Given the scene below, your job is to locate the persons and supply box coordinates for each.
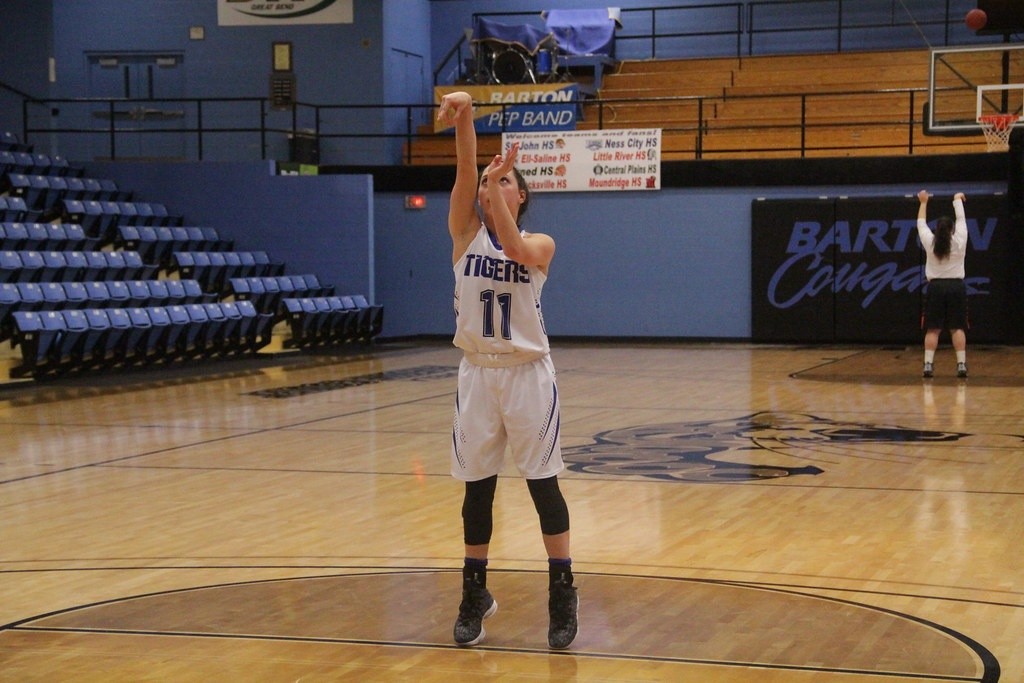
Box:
[434,91,579,647]
[918,190,968,378]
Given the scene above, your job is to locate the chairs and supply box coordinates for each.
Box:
[0,129,386,381]
[549,7,617,90]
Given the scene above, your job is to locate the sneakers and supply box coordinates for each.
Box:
[923,363,933,378]
[548,584,578,648]
[957,362,968,378]
[453,587,497,645]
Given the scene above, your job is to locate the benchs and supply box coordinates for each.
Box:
[399,49,1024,166]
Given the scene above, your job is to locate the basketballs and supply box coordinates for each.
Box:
[966,9,987,30]
